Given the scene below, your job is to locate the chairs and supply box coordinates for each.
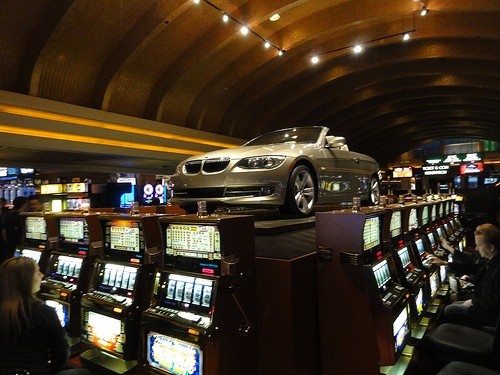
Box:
[428,313,500,375]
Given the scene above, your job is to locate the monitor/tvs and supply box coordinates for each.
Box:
[24,216,49,242]
[56,217,91,244]
[141,327,205,375]
[89,259,141,299]
[163,222,224,272]
[13,245,45,268]
[100,218,144,260]
[80,307,128,356]
[157,267,215,312]
[38,295,71,328]
[363,175,498,362]
[48,251,84,282]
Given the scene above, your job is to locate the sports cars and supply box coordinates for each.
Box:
[170,125,382,218]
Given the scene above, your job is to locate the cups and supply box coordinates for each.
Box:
[132,202,139,216]
[353,197,360,211]
[379,193,447,208]
[197,200,207,218]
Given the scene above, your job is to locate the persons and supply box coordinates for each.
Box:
[0,196,42,257]
[426,223,500,327]
[0,257,92,375]
